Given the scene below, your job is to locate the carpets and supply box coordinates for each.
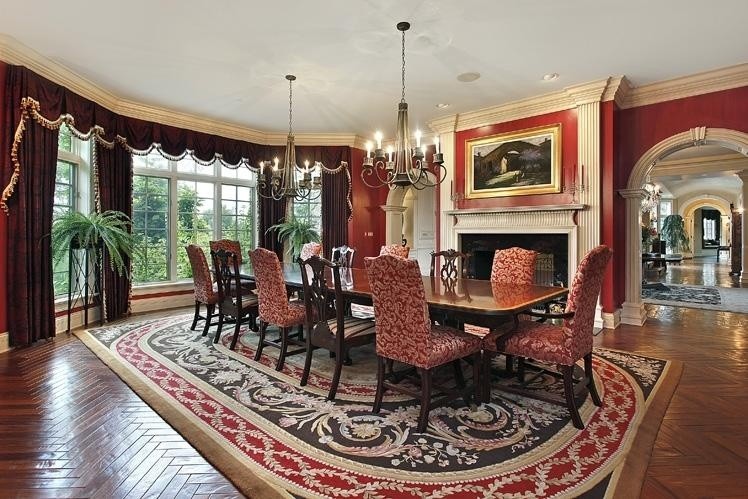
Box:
[641,282,748,314]
[72,311,684,499]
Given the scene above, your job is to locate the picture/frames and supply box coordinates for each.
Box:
[464,122,563,200]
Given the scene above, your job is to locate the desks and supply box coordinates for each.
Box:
[716,246,729,262]
[642,257,666,277]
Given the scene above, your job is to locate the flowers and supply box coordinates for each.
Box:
[649,228,657,236]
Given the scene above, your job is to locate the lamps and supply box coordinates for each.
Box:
[641,182,662,210]
[256,75,323,202]
[361,21,447,191]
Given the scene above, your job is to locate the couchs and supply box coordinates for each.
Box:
[664,253,682,266]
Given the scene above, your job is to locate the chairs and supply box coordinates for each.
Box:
[483,245,614,430]
[296,255,375,400]
[378,245,410,258]
[430,249,469,278]
[247,248,329,371]
[210,248,259,351]
[300,242,322,260]
[331,245,356,268]
[185,244,237,337]
[209,239,242,269]
[362,253,483,434]
[462,247,537,373]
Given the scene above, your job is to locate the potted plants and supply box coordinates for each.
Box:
[40,209,136,281]
